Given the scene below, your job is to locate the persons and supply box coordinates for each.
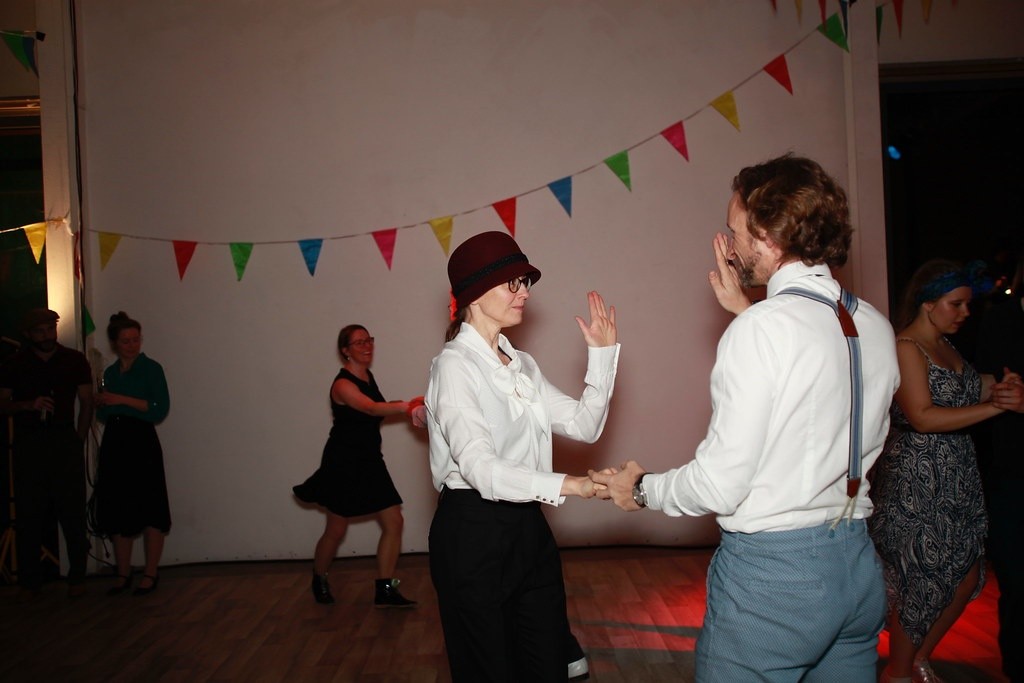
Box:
[292,324,429,609]
[0,311,172,602]
[870,258,1024,683]
[588,152,901,683]
[407,231,620,683]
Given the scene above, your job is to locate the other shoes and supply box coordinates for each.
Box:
[912,658,942,682]
[881,668,912,683]
[130,569,160,598]
[311,566,336,606]
[105,573,134,598]
[374,577,418,610]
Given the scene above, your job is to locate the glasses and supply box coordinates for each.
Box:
[348,336,375,349]
[508,276,532,293]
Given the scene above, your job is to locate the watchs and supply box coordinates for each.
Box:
[632,472,654,508]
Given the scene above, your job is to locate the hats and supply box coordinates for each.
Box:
[447,231,542,310]
